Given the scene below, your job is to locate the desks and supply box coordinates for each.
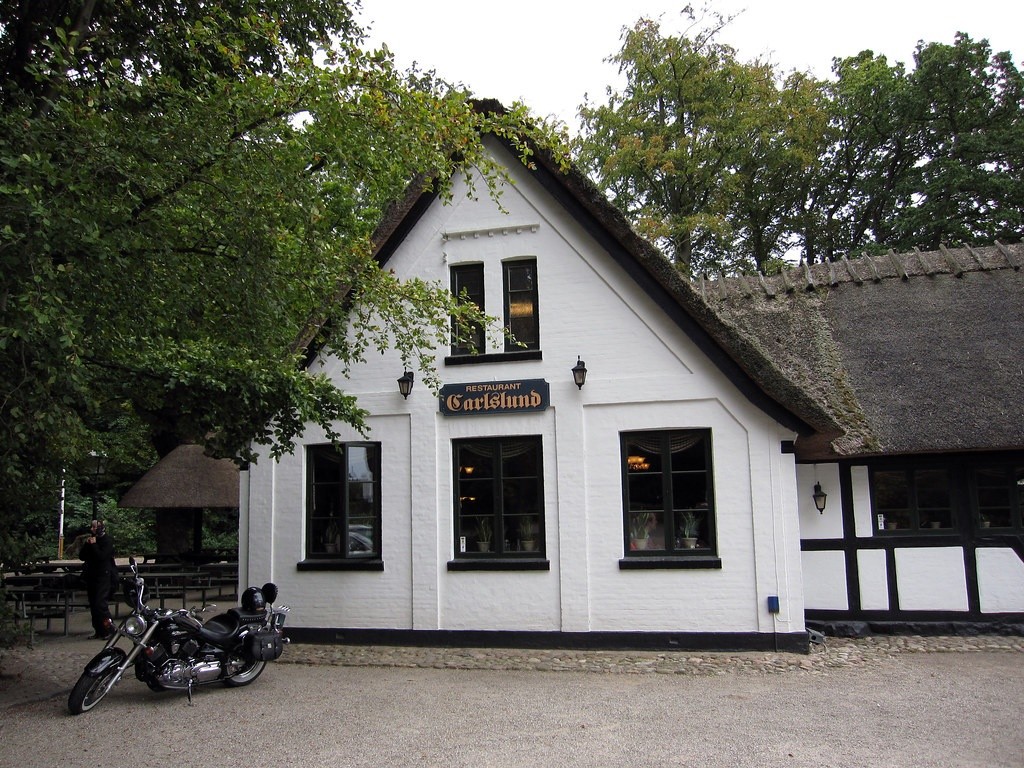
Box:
[4,588,75,642]
[116,563,182,573]
[118,570,211,609]
[200,562,238,601]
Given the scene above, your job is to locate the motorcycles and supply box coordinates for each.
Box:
[68,556,291,715]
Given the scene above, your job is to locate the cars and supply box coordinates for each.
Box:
[349,524,375,559]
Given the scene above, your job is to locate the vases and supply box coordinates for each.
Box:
[929,521,941,528]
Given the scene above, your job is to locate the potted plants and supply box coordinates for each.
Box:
[630,512,652,550]
[321,518,339,553]
[980,514,992,528]
[472,516,493,552]
[885,512,899,529]
[680,511,701,549]
[518,516,538,551]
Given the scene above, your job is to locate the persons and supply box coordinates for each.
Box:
[79,519,120,640]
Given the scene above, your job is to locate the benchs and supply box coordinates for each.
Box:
[0,553,239,641]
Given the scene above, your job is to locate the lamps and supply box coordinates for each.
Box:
[627,456,650,472]
[571,355,588,390]
[811,481,827,514]
[397,367,415,400]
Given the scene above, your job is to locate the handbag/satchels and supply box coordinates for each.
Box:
[244,629,283,662]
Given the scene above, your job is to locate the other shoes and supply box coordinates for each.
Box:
[88,633,102,640]
[103,632,115,640]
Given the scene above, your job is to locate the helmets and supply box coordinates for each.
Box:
[241,587,266,615]
[123,582,150,608]
